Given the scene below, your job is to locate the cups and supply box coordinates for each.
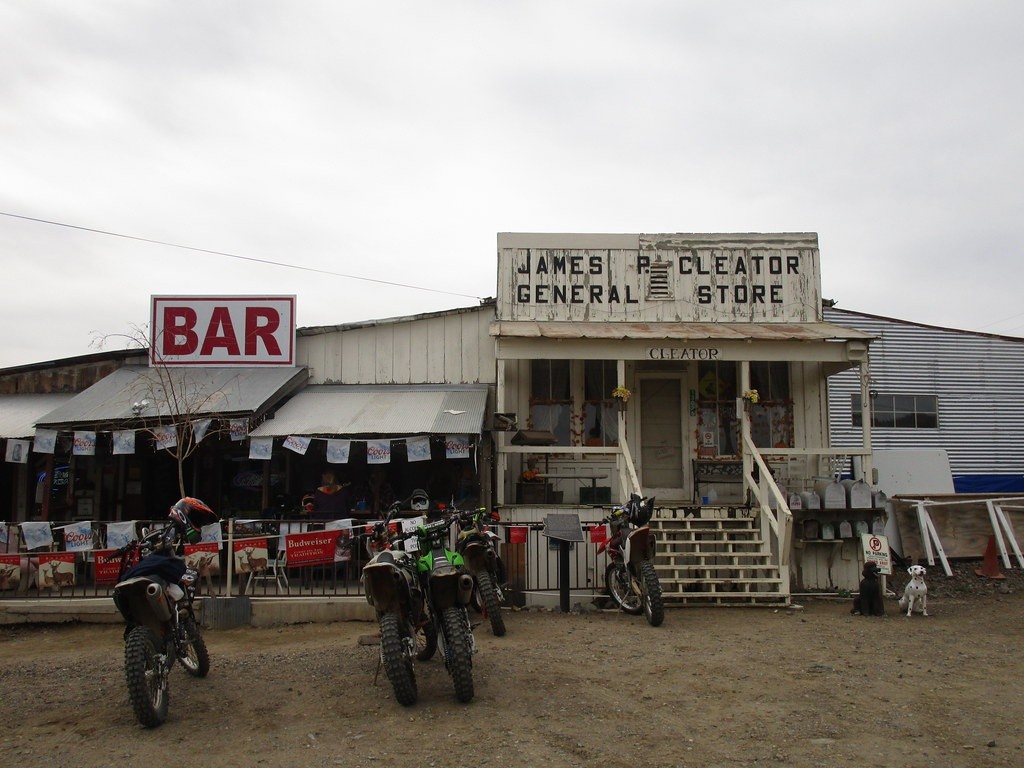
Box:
[702,497,709,504]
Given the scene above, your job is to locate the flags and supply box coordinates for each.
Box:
[406,436,431,462]
[445,434,469,458]
[65,522,93,551]
[21,522,52,550]
[249,436,391,464]
[277,519,425,553]
[200,523,223,550]
[5,417,248,464]
[106,521,132,549]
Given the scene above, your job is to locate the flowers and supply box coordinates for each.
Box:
[742,389,760,403]
[517,467,545,484]
[613,384,631,401]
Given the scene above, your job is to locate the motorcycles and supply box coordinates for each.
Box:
[355,505,507,705]
[102,519,209,730]
[149,627,151,628]
[596,494,666,627]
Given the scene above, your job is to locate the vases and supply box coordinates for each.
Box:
[618,399,627,411]
[579,487,611,505]
[516,483,554,504]
[744,399,752,413]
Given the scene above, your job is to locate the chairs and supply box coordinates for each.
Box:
[247,550,290,597]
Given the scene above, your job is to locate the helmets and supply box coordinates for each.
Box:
[302,494,316,511]
[410,489,430,511]
[275,492,295,512]
[335,534,349,548]
[168,496,217,546]
[630,494,656,529]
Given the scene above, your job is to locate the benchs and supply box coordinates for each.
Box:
[692,458,776,507]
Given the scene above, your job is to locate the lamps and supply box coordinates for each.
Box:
[132,399,150,414]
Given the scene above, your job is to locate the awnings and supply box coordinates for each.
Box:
[0,390,79,442]
[29,364,308,421]
[245,384,488,442]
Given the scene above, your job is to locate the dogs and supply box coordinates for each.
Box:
[851,560,884,616]
[898,565,931,617]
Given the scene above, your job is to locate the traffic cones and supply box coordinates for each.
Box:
[974,534,1007,580]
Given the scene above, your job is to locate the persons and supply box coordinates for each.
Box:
[312,471,356,519]
[457,463,473,496]
[371,471,397,510]
[586,428,603,446]
